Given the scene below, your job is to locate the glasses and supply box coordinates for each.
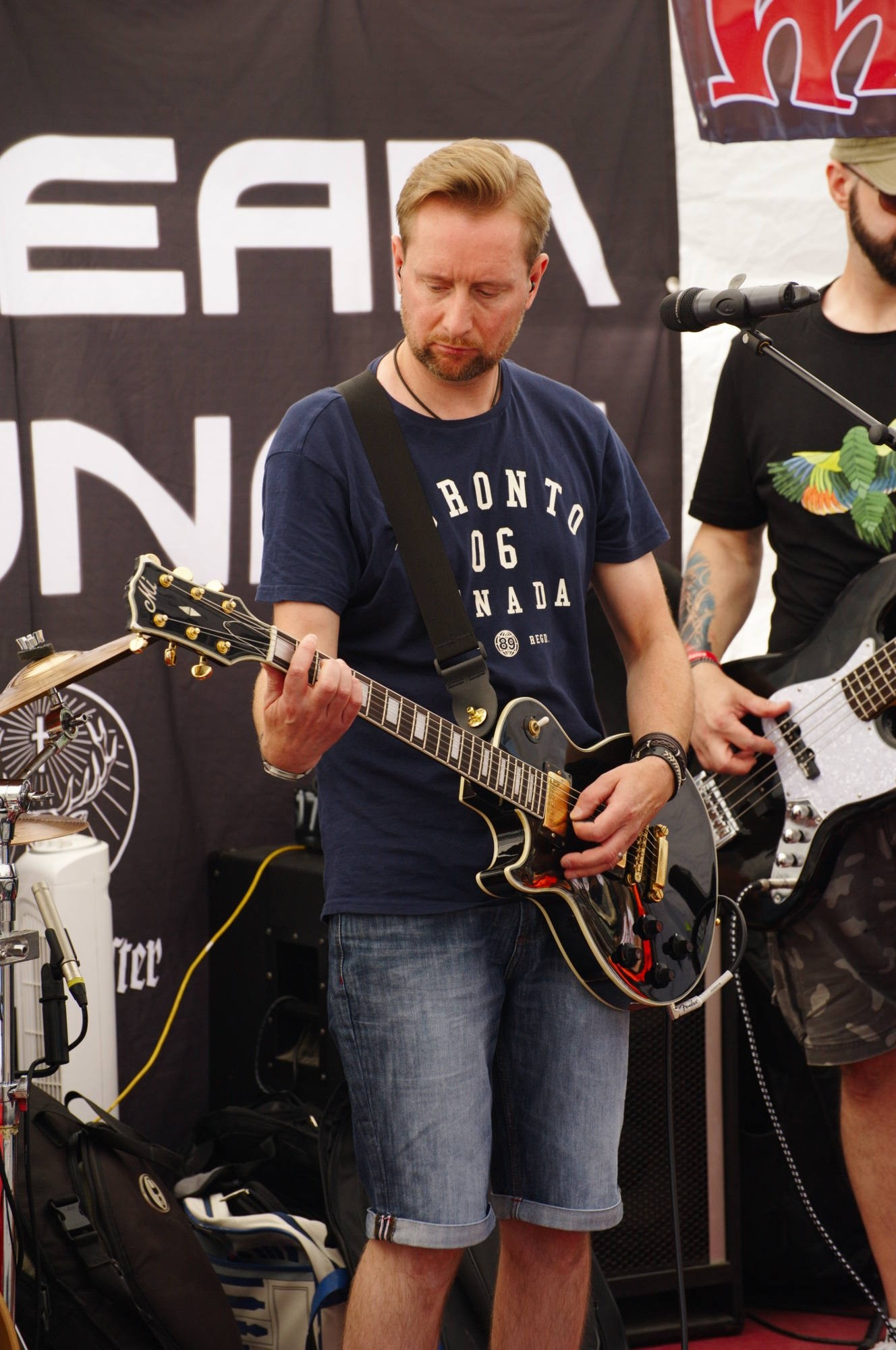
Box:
[840,163,896,215]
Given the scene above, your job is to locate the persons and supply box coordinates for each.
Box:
[255,139,702,1350]
[680,133,896,1350]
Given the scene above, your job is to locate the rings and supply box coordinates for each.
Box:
[617,851,624,860]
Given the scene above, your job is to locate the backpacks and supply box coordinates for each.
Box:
[170,1163,351,1350]
[8,1080,245,1350]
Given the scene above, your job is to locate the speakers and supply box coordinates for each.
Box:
[206,841,742,1299]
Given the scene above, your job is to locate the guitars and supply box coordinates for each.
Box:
[122,549,723,1005]
[713,532,896,934]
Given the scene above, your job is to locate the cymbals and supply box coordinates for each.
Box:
[9,811,87,846]
[2,629,157,714]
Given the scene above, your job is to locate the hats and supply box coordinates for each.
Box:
[828,137,896,197]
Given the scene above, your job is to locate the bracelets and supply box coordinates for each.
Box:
[688,649,720,666]
[632,733,686,802]
[263,758,317,782]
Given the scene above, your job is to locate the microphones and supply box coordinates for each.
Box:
[30,881,89,1008]
[658,281,822,334]
